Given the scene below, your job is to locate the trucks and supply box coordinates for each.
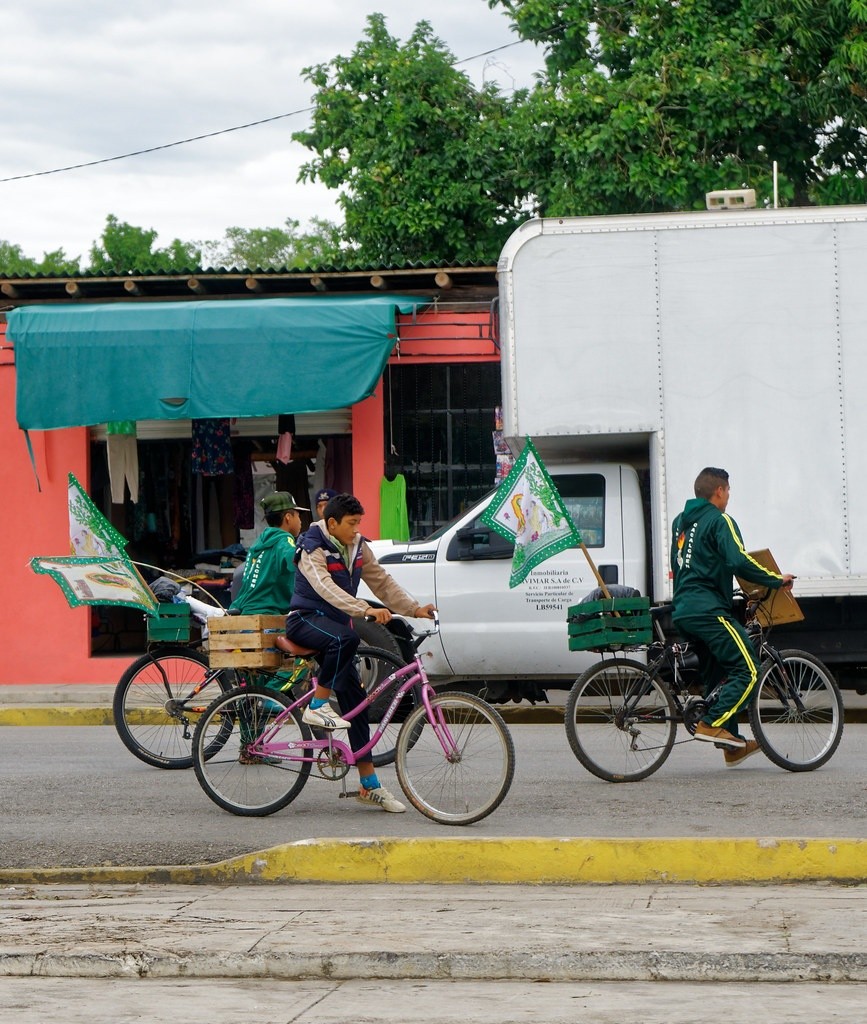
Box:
[286,188,866,721]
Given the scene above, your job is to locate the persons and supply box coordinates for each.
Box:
[228,491,311,765]
[284,492,440,813]
[315,488,337,519]
[670,467,795,767]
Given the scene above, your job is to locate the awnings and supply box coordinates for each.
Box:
[2,287,439,494]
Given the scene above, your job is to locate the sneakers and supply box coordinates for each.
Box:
[301,703,352,729]
[695,721,762,768]
[238,746,282,764]
[356,783,406,813]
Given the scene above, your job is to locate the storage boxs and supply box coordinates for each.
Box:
[206,614,289,667]
[567,596,651,651]
[146,604,191,641]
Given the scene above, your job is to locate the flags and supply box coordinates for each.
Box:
[29,556,161,621]
[479,436,583,590]
[67,471,130,558]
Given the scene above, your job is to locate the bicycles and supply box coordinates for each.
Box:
[111,643,425,772]
[190,608,517,826]
[562,575,847,783]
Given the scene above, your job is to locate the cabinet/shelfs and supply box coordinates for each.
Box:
[384,408,498,527]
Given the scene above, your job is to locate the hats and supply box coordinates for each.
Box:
[315,489,337,503]
[261,491,311,513]
